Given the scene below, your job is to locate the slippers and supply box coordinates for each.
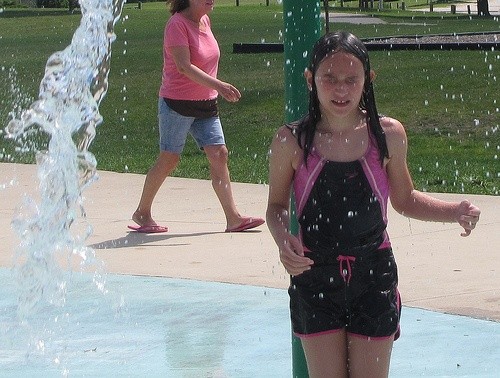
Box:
[127,225,168,232]
[224,215,266,232]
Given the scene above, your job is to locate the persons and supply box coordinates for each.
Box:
[265,30,480,378]
[127,0,264,234]
